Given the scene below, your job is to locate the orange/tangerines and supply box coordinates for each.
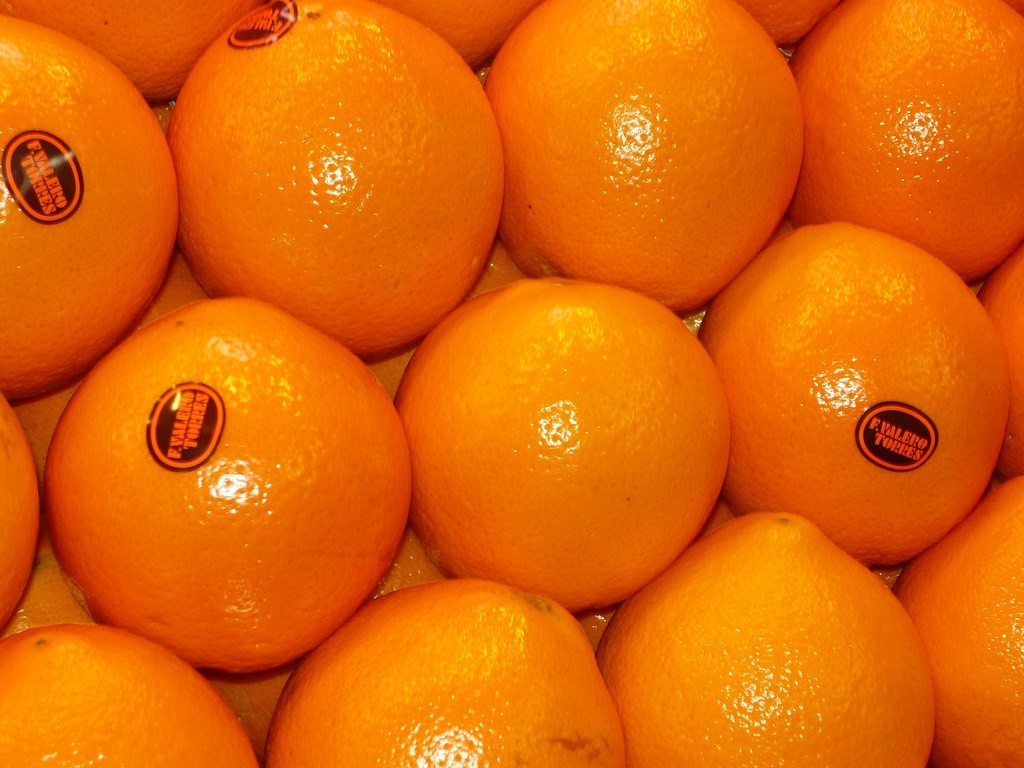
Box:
[0,0,1024,768]
